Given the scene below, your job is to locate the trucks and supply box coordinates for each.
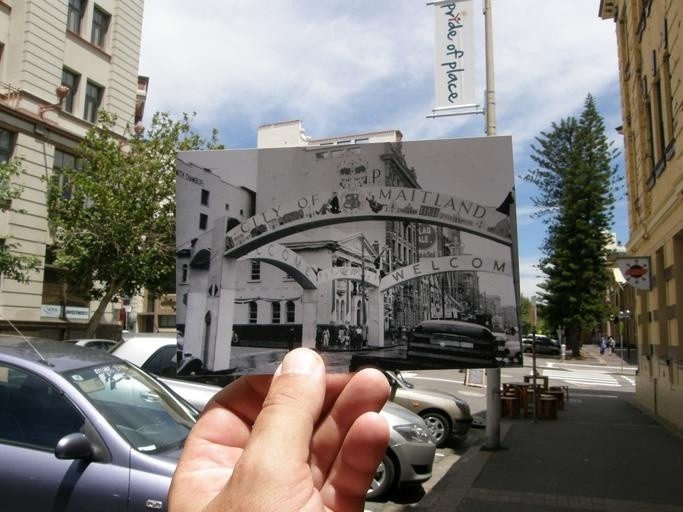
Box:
[348,322,510,371]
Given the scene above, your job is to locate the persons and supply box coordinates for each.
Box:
[318,324,362,346]
[598,334,616,357]
[165,348,390,512]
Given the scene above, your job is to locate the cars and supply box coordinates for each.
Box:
[523,333,559,355]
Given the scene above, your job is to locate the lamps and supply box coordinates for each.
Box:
[119,123,145,149]
[40,86,69,127]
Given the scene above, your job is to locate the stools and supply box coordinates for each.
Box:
[502,375,569,419]
